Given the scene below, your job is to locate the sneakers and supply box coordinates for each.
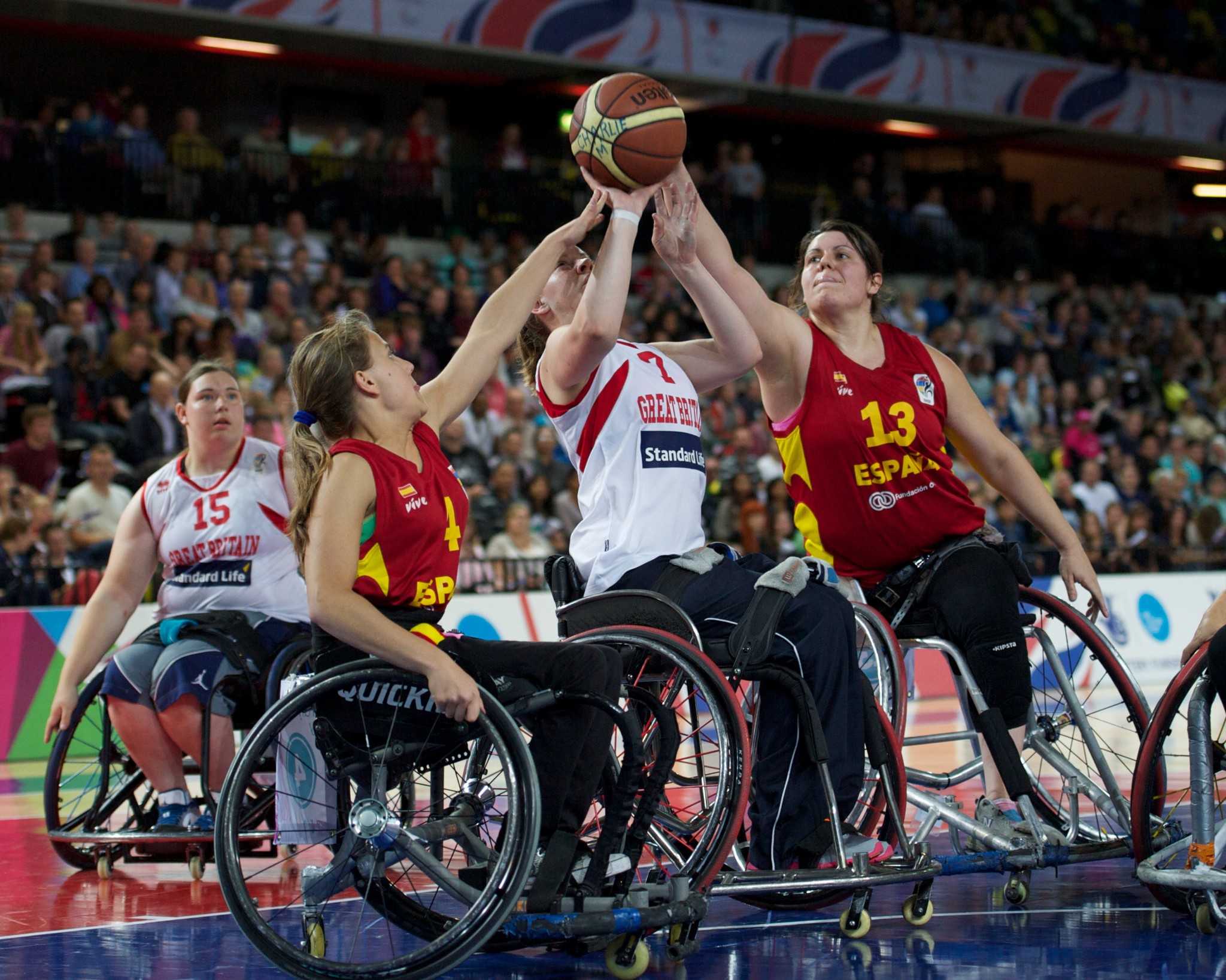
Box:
[486,846,631,892]
[968,793,1068,854]
[746,832,895,872]
[153,787,192,833]
[191,804,218,831]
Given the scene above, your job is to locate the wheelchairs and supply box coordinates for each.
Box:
[213,622,751,980]
[460,549,1226,980]
[44,612,312,881]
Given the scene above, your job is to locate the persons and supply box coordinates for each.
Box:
[1178,588,1226,663]
[289,187,606,903]
[660,159,1108,848]
[0,98,1226,589]
[518,166,892,866]
[44,364,311,831]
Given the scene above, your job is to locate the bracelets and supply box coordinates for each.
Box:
[609,209,640,226]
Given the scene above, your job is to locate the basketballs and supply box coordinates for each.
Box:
[568,73,687,191]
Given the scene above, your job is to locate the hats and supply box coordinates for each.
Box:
[1075,409,1093,423]
